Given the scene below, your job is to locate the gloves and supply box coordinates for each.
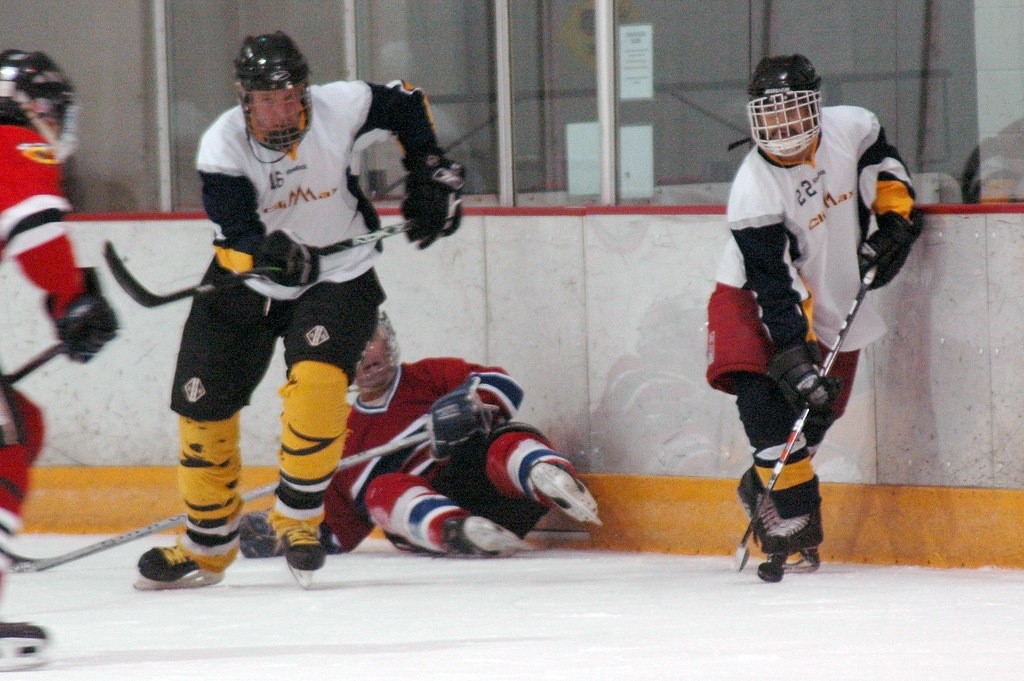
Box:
[426,386,490,460]
[859,211,923,290]
[44,267,119,363]
[400,147,465,250]
[253,228,320,288]
[767,345,842,425]
[239,507,286,558]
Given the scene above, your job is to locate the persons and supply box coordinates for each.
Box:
[240,311,598,557]
[961,118,1024,205]
[706,54,922,554]
[0,46,118,656]
[137,30,467,582]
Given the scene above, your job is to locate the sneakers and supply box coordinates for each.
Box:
[735,463,824,583]
[0,621,47,671]
[267,510,324,587]
[133,542,225,590]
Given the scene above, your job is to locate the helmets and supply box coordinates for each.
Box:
[234,30,312,147]
[747,54,821,156]
[0,50,74,126]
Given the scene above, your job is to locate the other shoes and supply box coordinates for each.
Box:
[530,463,604,526]
[447,516,535,557]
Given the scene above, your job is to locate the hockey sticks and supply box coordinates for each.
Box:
[103,216,407,308]
[735,261,880,572]
[1,333,74,391]
[0,402,485,575]
